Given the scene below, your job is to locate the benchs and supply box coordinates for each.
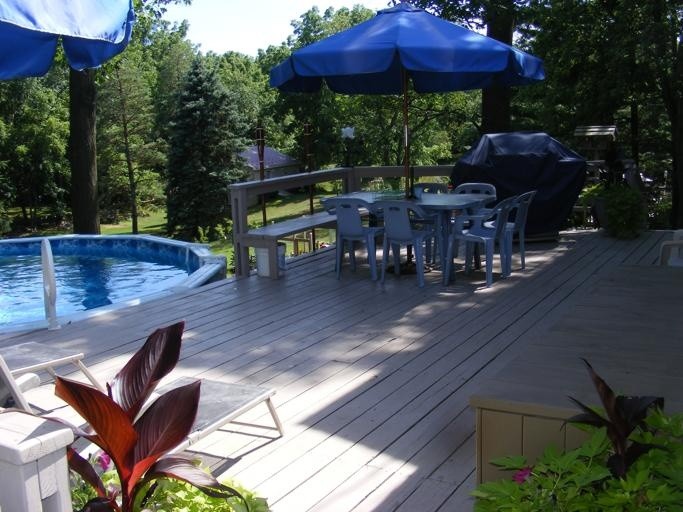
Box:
[238,207,370,280]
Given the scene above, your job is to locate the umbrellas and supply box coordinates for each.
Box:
[0,1,135,84]
[267,1,545,196]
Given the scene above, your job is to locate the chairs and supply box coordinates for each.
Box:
[0,341,284,458]
[319,183,537,289]
[658,229,683,267]
